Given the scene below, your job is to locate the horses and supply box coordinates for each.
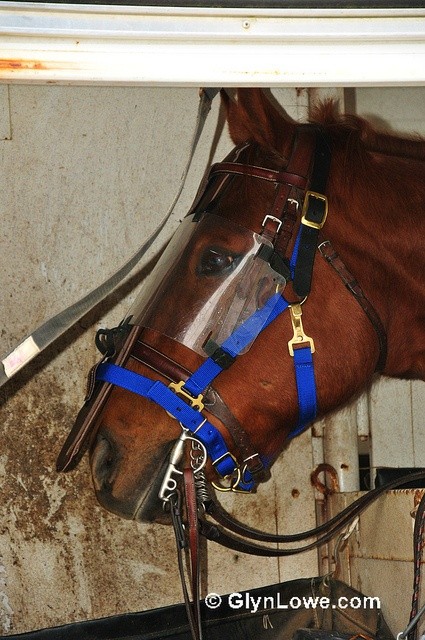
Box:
[84,86,425,527]
